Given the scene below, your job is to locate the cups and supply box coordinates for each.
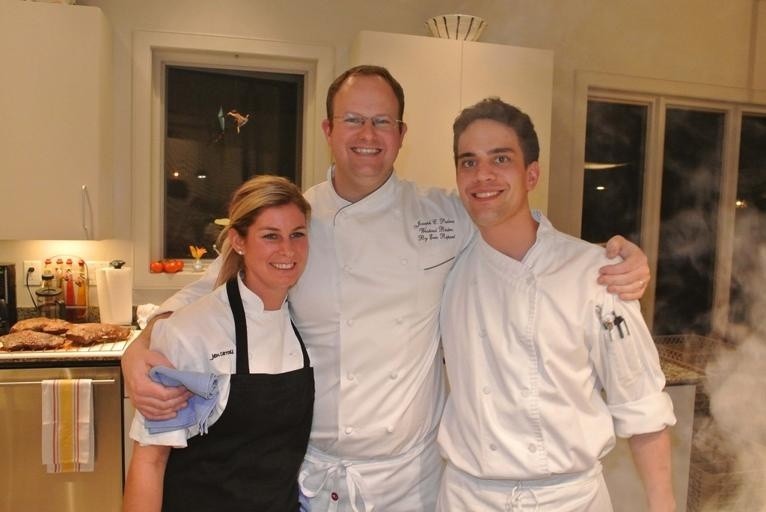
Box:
[36,287,67,320]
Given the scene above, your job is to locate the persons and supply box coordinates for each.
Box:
[118,63,652,511]
[118,174,316,510]
[434,96,680,511]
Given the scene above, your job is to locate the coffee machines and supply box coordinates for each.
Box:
[0,261,18,334]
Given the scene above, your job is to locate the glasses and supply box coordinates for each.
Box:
[331,110,403,130]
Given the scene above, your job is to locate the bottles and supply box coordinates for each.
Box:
[44,258,89,318]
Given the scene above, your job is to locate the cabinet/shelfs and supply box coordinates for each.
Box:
[0,358,136,510]
[0,2,116,243]
[334,29,555,218]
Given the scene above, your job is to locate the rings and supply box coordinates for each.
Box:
[638,278,646,289]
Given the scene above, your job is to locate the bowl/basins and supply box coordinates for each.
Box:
[423,13,489,42]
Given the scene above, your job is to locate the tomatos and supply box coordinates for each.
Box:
[164,261,178,273]
[173,260,184,270]
[151,261,163,273]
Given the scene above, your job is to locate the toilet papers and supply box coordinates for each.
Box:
[95,266,134,326]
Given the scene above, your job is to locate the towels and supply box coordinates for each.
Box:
[40,377,95,475]
